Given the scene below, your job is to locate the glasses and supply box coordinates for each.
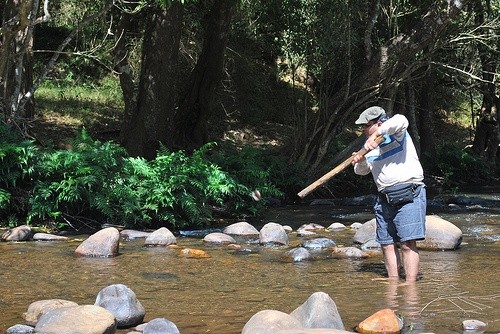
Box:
[359,122,371,128]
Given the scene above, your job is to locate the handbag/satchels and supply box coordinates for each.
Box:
[379,184,418,206]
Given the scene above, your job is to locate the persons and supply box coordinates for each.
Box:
[352,106,426,285]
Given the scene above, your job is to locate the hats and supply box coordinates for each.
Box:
[355,106,386,124]
